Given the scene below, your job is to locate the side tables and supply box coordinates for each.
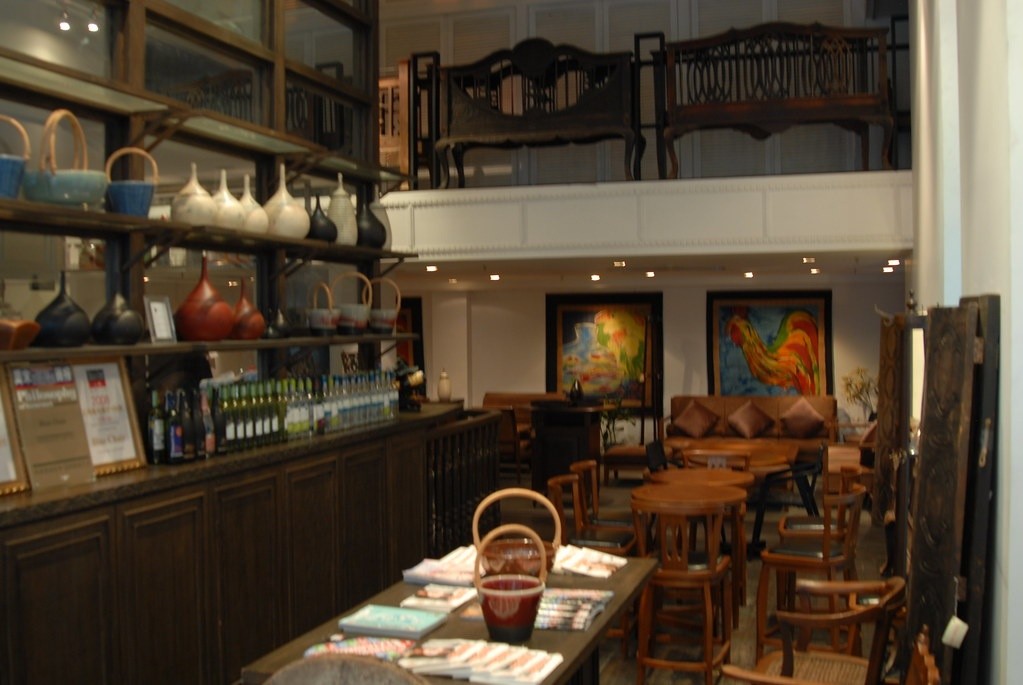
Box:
[840,433,861,443]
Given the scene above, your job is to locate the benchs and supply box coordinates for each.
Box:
[437,37,636,189]
[664,20,894,181]
[658,395,837,463]
[483,392,544,440]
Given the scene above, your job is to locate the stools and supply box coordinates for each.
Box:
[604,444,674,490]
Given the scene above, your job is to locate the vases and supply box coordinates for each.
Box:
[570,379,582,406]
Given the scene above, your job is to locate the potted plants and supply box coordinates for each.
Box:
[841,364,878,422]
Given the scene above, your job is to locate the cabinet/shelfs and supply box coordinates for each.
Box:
[532,404,604,507]
[0,196,419,358]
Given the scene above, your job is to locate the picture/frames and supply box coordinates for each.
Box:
[0,360,30,494]
[5,356,147,485]
[546,291,664,416]
[706,288,833,396]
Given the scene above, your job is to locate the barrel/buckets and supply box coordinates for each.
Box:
[303,271,401,337]
[472,488,562,577]
[472,523,547,643]
[0,106,160,219]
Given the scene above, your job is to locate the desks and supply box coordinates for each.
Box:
[240,556,660,685]
[629,484,748,629]
[677,441,799,512]
[651,467,756,488]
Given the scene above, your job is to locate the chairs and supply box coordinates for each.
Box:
[465,407,530,487]
[546,442,907,685]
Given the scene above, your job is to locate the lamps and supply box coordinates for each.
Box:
[60,5,71,32]
[87,6,99,34]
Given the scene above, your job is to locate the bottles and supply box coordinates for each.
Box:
[34,257,266,347]
[170,162,393,252]
[437,368,451,402]
[147,368,401,465]
[570,379,585,403]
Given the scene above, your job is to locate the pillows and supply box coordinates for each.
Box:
[672,399,719,438]
[779,397,823,438]
[728,398,773,439]
